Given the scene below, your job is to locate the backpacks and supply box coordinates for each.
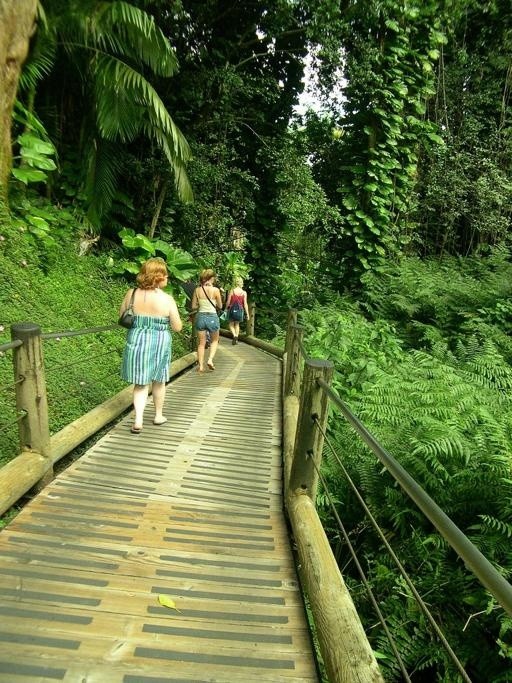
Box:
[230,301,244,321]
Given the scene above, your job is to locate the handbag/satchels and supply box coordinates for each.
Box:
[118,309,133,327]
[216,305,223,317]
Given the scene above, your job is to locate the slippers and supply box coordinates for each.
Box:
[153,417,166,425]
[131,425,141,433]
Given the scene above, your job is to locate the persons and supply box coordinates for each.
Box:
[119,257,183,433]
[225,276,249,345]
[192,269,223,372]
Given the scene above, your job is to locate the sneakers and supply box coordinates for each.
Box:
[232,337,238,345]
